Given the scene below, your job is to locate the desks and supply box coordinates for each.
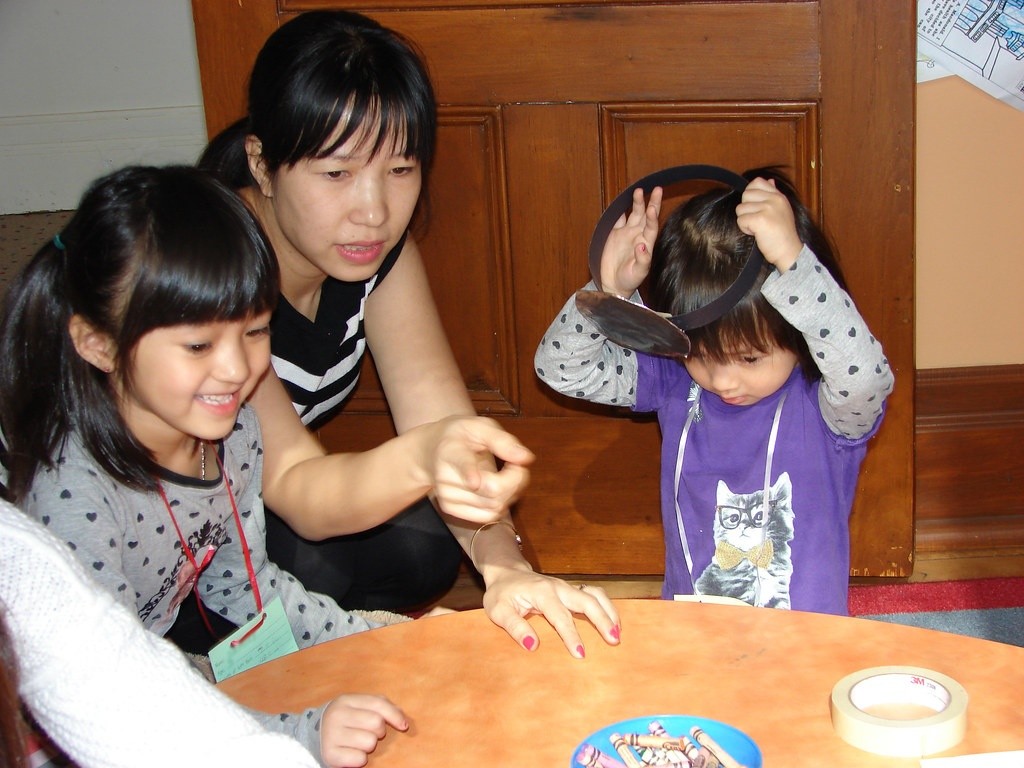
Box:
[213,600,1023,767]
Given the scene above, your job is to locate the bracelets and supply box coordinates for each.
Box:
[469,520,523,565]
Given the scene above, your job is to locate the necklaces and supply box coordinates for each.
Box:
[201,442,205,481]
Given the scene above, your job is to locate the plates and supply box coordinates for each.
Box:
[571,715,762,768]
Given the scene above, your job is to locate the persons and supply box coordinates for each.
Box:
[195,10,620,657]
[532,162,893,616]
[0,163,459,768]
[0,499,321,768]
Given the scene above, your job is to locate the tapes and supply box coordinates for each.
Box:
[830,666,971,759]
